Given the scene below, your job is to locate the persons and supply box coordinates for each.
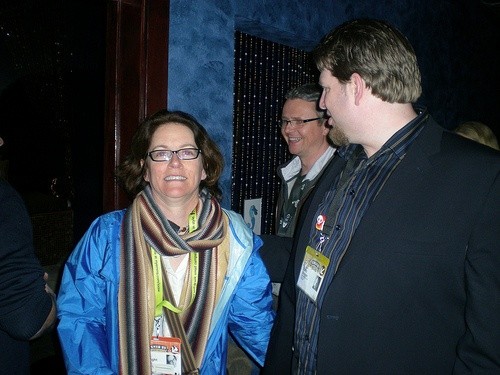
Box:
[0,136,56,375]
[261,17,500,375]
[276,83,337,238]
[454,121,499,150]
[55,110,276,375]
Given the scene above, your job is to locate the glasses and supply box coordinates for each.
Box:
[282,116,321,128]
[146,147,201,163]
